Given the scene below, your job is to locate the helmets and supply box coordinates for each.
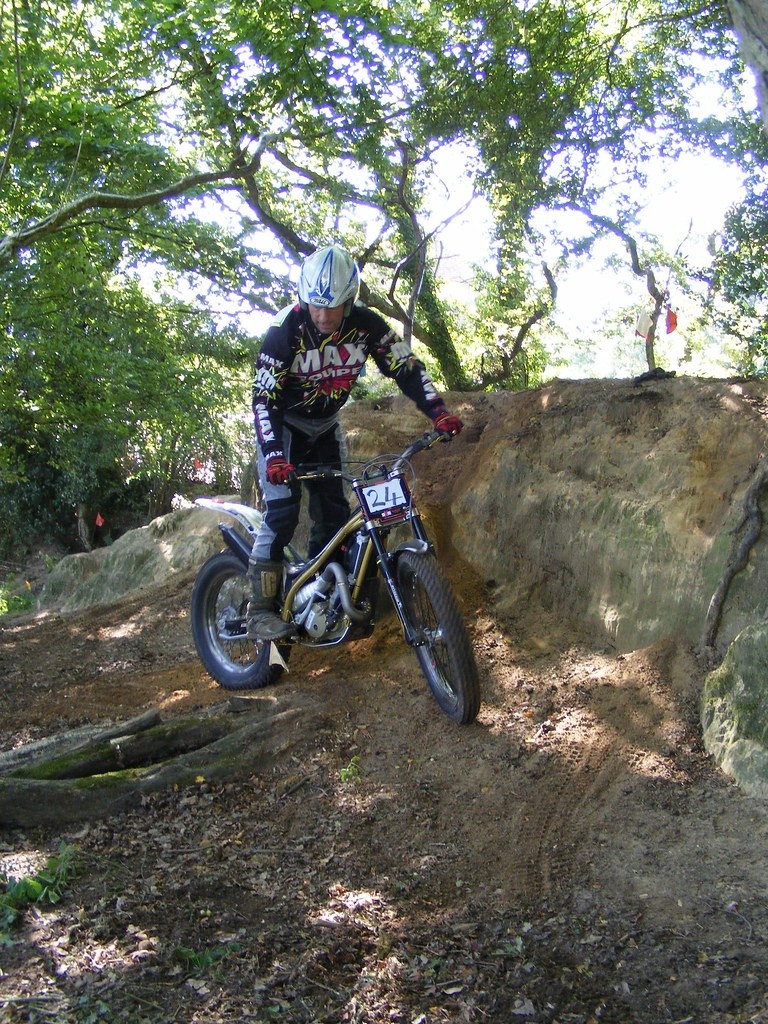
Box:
[297,246,360,317]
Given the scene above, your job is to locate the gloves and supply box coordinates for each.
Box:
[432,411,464,441]
[267,457,297,486]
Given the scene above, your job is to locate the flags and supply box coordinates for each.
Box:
[26,580,31,591]
[635,309,653,339]
[665,308,678,333]
[194,457,204,469]
[96,513,104,527]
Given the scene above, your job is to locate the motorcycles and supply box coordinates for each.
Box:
[191,420,481,725]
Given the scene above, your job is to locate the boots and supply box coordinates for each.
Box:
[246,562,294,640]
[314,602,375,644]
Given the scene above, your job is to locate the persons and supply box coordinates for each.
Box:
[245,244,462,642]
